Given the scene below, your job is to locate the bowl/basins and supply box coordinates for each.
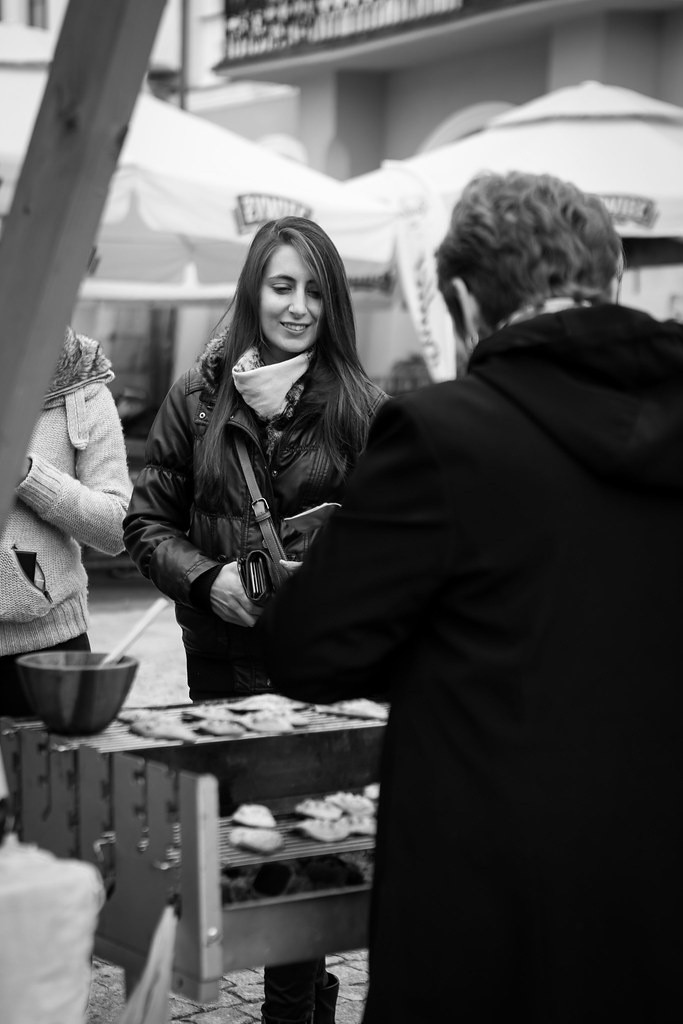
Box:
[16,650,138,739]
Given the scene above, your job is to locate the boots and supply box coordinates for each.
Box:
[261,971,340,1024]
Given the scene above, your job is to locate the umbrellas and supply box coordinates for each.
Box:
[1,69,682,287]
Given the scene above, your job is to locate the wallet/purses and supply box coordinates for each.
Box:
[236,550,282,607]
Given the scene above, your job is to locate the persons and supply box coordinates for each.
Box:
[246,167,683,1024]
[0,325,136,719]
[122,217,391,1023]
